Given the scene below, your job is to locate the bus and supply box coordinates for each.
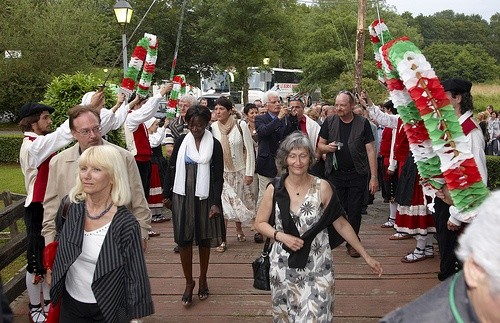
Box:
[199,66,305,107]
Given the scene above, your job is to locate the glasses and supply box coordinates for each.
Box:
[289,97,303,104]
[338,91,354,101]
[73,125,101,135]
[265,101,280,104]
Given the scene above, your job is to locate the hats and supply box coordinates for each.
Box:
[20,102,55,119]
[441,79,473,92]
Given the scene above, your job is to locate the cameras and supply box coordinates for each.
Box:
[287,107,292,112]
[331,141,343,150]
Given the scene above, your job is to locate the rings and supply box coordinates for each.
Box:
[216,216,217,217]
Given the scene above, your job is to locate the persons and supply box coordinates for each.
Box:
[289,98,336,176]
[231,100,268,157]
[378,192,500,323]
[319,93,378,258]
[81,82,198,252]
[476,105,500,156]
[354,79,488,281]
[198,98,208,107]
[163,104,226,306]
[255,132,383,323]
[45,145,155,323]
[212,96,255,251]
[18,90,103,323]
[254,89,300,244]
[41,105,152,253]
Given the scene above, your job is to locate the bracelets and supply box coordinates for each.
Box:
[274,231,279,242]
[372,176,377,178]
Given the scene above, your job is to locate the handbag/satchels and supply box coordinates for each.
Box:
[243,145,256,162]
[252,252,270,291]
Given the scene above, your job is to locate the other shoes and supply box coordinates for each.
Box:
[217,241,227,253]
[174,246,178,251]
[237,233,245,242]
[147,230,160,237]
[425,254,435,258]
[254,233,263,242]
[28,311,46,323]
[389,235,410,240]
[381,224,394,228]
[400,254,425,262]
[42,305,49,317]
[149,217,170,223]
[347,245,360,257]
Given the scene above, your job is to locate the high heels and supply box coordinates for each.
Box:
[182,280,195,304]
[198,278,208,300]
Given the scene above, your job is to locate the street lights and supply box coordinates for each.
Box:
[113,0,134,78]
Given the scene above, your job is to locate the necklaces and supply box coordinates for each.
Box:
[297,193,299,195]
[86,201,114,219]
